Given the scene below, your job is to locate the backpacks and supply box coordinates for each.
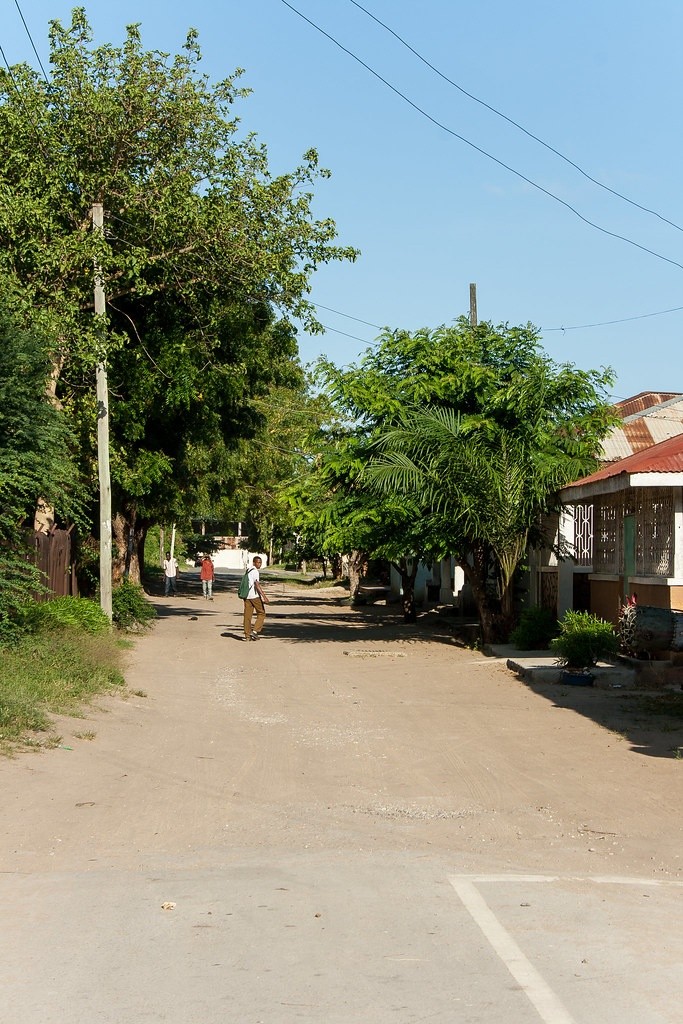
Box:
[238,568,255,599]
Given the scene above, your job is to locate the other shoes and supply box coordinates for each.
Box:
[246,638,251,641]
[250,632,260,640]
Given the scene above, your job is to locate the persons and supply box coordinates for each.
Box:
[243,555,269,642]
[196,551,215,600]
[163,551,179,597]
